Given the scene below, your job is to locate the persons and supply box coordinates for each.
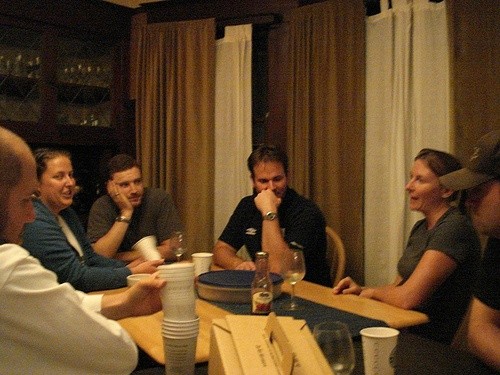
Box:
[86,154,183,261]
[212,145,333,289]
[0,127,198,375]
[332,148,481,347]
[438,130,500,375]
[20,147,165,293]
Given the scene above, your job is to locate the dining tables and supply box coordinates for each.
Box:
[88,259,431,366]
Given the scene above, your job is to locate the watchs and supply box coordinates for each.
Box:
[262,211,279,221]
[115,215,131,224]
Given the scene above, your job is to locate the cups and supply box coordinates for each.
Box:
[156,264,197,321]
[160,316,201,375]
[131,235,161,263]
[191,252,214,278]
[359,327,400,375]
[312,321,356,375]
[126,273,151,290]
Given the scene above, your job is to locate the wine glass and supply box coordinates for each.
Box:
[168,231,190,264]
[279,249,308,312]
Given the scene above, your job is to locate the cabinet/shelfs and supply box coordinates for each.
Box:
[0,0,136,232]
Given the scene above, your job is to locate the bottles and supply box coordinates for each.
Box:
[250,251,274,316]
[78,105,99,127]
[0,53,110,87]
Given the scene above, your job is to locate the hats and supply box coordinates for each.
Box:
[438,128,500,189]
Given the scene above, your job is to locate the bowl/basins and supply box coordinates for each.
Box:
[196,269,284,304]
